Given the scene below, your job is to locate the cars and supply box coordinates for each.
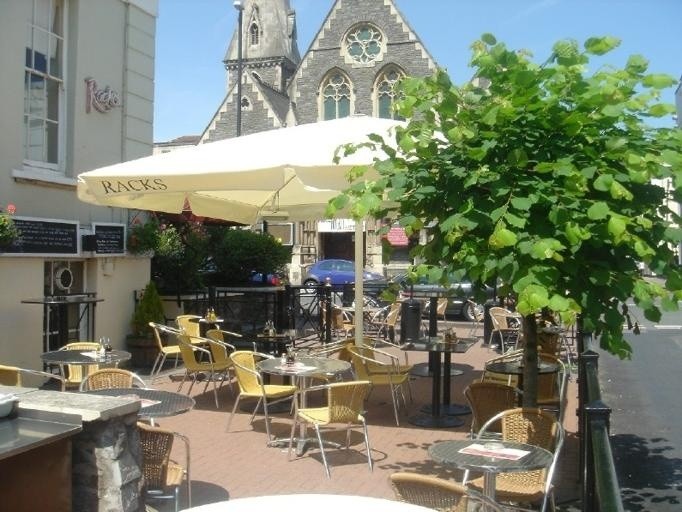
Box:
[300,259,390,298]
[197,255,291,292]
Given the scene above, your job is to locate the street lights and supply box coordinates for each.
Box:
[232,0,246,138]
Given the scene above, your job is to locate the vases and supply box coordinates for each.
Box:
[0,237,16,252]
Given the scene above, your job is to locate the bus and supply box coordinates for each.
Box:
[344,262,514,323]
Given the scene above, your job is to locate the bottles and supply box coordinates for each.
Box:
[95,336,113,358]
[206,308,215,321]
[443,327,457,343]
[280,344,294,364]
[263,320,276,337]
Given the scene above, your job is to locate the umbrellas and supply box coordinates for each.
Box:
[72,115,455,350]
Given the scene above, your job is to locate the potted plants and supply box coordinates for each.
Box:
[125,281,169,370]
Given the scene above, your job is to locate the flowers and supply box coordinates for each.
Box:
[0,203,24,240]
[125,216,187,259]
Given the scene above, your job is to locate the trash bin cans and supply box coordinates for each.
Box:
[482,301,502,347]
[399,299,420,345]
